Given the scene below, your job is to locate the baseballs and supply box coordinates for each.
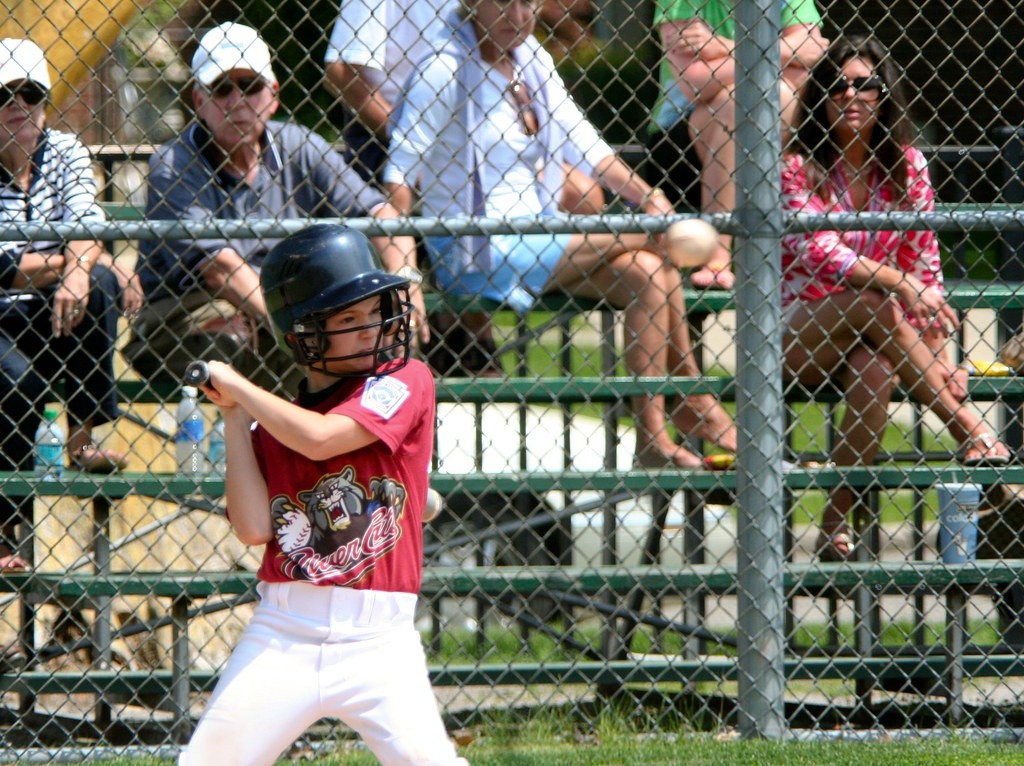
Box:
[666,219,718,270]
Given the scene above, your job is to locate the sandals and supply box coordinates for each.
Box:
[964,430,1011,463]
[815,521,855,560]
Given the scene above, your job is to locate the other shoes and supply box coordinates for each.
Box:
[71,446,127,472]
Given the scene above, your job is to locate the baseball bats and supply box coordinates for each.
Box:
[184,359,444,523]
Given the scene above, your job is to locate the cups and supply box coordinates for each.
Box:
[936,483,982,564]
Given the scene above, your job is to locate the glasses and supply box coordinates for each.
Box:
[202,77,270,96]
[823,76,881,97]
[508,79,539,137]
[0,81,46,106]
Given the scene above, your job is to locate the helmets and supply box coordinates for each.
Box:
[261,226,411,343]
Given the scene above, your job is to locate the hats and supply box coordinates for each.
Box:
[193,21,277,89]
[1,38,51,90]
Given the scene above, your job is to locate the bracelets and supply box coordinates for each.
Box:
[66,256,94,268]
[392,267,423,283]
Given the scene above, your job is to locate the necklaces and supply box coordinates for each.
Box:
[509,62,523,92]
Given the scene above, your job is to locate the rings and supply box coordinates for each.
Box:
[928,317,935,323]
[409,320,417,327]
[74,309,79,315]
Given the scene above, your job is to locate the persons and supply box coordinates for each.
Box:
[782,35,1011,557]
[650,0,828,288]
[0,38,144,573]
[124,22,443,521]
[175,225,468,766]
[382,0,736,469]
[326,0,506,377]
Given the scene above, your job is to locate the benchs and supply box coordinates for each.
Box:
[0,144,1022,761]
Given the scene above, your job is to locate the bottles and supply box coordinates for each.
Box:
[210,409,226,479]
[34,409,64,481]
[177,385,203,478]
